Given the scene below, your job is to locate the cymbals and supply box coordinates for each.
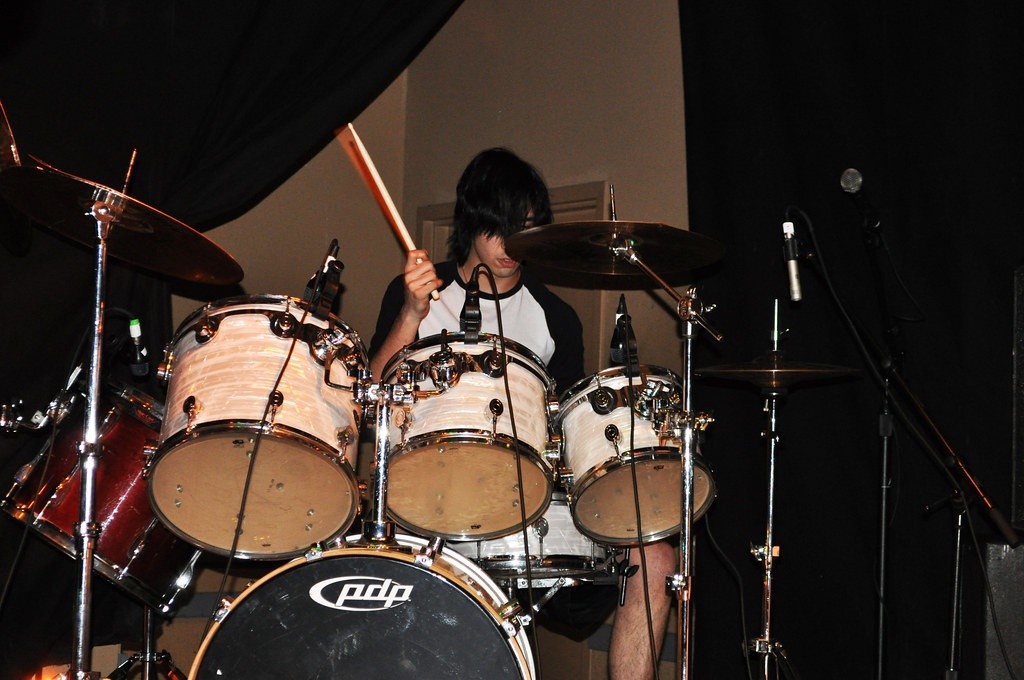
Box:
[504,220,726,276]
[691,349,853,388]
[1,163,246,287]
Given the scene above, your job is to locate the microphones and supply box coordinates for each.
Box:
[610,294,637,362]
[783,212,802,301]
[129,315,149,376]
[460,268,482,332]
[303,243,339,303]
[841,167,882,233]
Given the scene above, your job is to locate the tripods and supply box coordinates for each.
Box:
[104,602,187,680]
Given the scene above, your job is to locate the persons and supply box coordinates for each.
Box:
[367,147,678,680]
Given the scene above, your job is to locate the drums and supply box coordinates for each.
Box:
[437,491,622,589]
[557,365,718,546]
[381,331,553,543]
[182,532,537,680]
[145,293,372,562]
[0,373,202,616]
